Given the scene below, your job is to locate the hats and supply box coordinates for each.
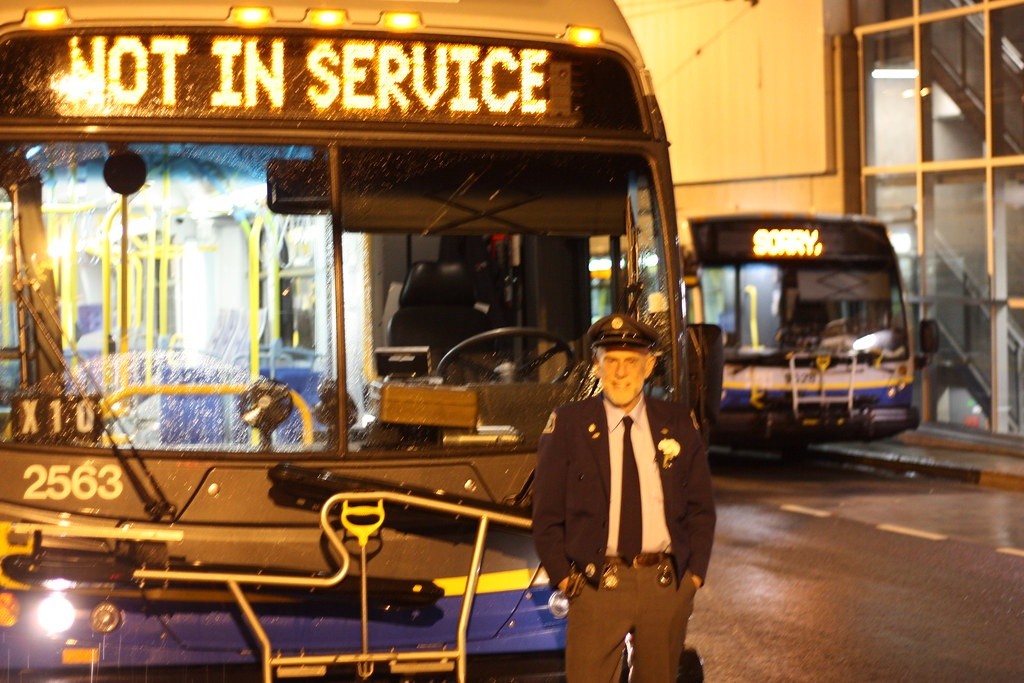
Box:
[587,313,659,349]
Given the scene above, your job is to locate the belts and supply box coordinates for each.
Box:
[605,552,672,566]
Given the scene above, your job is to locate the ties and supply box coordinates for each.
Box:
[617,416,642,566]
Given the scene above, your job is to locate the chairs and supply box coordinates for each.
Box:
[387,261,497,369]
[791,294,829,334]
[63,336,327,444]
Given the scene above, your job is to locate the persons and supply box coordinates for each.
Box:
[534,312,717,683]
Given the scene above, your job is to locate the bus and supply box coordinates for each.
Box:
[587,206,941,463]
[1,0,725,683]
